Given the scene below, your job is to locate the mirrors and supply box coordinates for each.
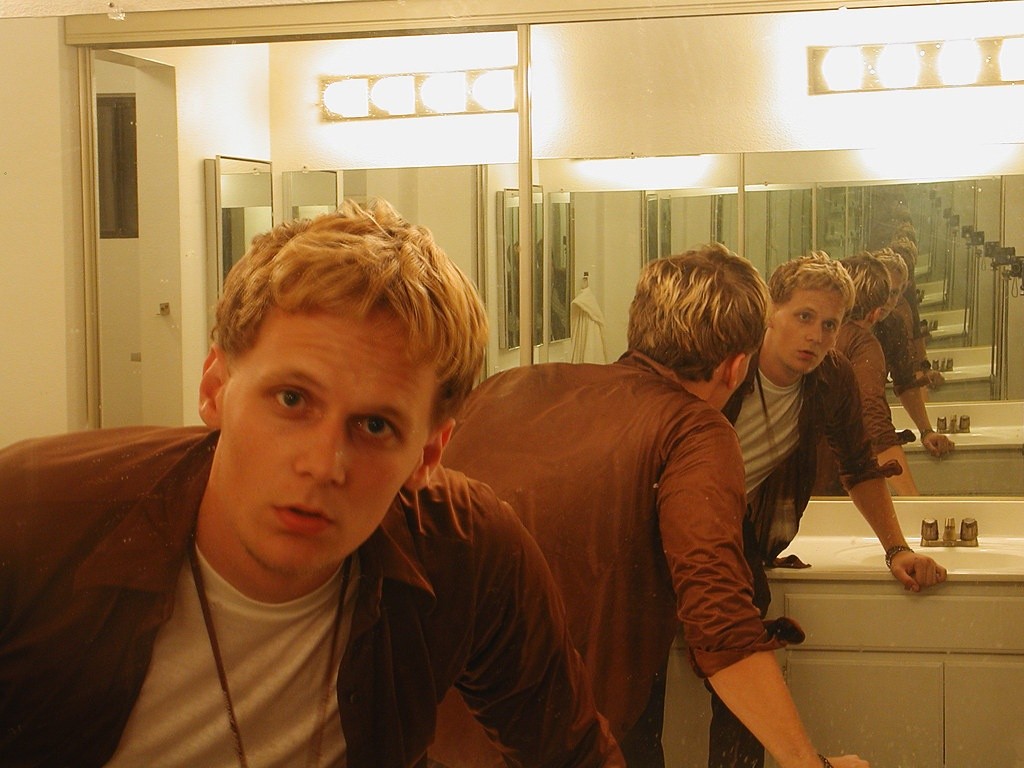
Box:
[205,154,274,353]
[497,188,521,351]
[525,0,1024,550]
[812,174,1007,386]
[861,183,957,306]
[844,180,978,337]
[334,165,487,389]
[661,196,672,259]
[742,141,1024,424]
[282,168,338,223]
[531,183,545,347]
[548,189,572,343]
[642,192,660,271]
[61,0,524,431]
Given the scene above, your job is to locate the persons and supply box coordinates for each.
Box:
[814,228,956,501]
[437,237,881,768]
[0,198,622,768]
[625,241,946,768]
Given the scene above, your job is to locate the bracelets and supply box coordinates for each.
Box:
[817,752,833,768]
[921,429,934,443]
[886,545,914,568]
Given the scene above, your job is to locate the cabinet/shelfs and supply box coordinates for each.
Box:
[664,581,1024,768]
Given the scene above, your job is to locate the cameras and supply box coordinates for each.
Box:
[928,189,1024,278]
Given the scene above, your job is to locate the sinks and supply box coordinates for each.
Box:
[924,328,956,335]
[896,432,1016,445]
[829,541,1024,577]
[933,369,985,377]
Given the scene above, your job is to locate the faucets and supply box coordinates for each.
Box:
[916,288,922,301]
[944,514,958,547]
[942,358,948,370]
[950,415,958,432]
[928,321,933,329]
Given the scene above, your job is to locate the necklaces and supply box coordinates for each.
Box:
[186,532,353,768]
[753,365,811,542]
